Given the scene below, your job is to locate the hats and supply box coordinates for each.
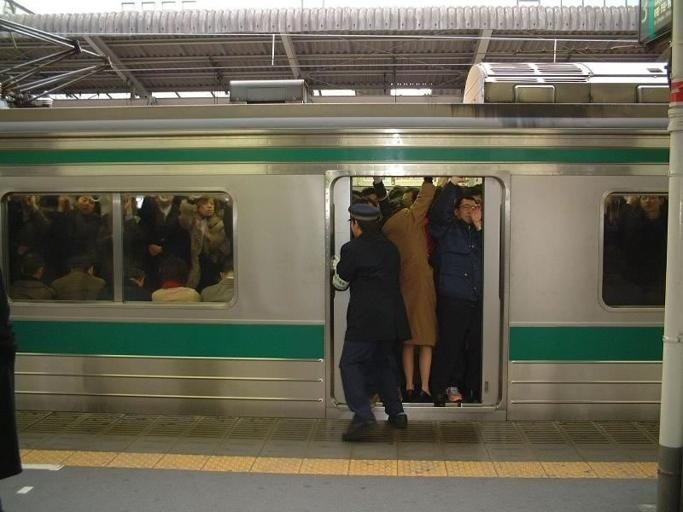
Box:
[347,204,380,221]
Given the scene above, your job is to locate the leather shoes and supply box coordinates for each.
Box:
[406,390,444,407]
[388,415,407,428]
[342,420,376,440]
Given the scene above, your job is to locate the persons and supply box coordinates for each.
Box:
[604,193,667,305]
[328,175,483,442]
[7,194,235,304]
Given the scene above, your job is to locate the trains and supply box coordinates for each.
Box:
[0,60,670,420]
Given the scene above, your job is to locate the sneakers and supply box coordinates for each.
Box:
[448,387,462,402]
